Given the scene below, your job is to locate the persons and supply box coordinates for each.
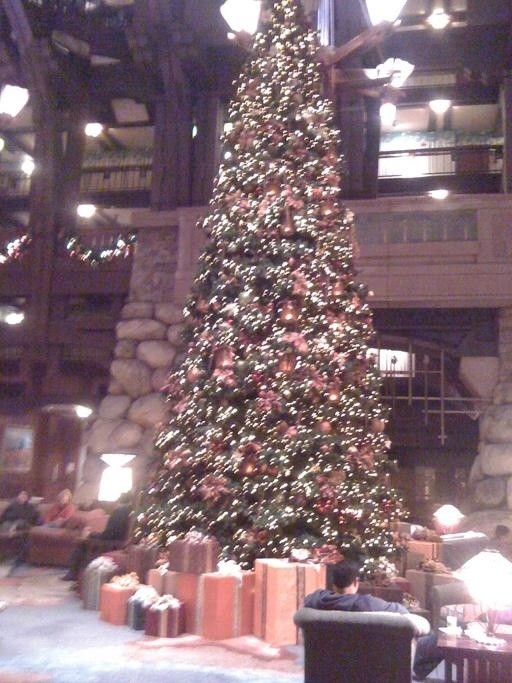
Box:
[305,558,446,682]
[58,492,134,590]
[412,525,434,540]
[11,489,75,566]
[0,489,40,526]
[489,525,511,560]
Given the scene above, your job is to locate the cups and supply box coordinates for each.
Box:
[445,615,458,640]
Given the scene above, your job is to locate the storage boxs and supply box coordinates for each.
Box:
[84,536,326,650]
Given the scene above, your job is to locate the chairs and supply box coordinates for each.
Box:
[29,508,110,565]
[294,582,511,681]
